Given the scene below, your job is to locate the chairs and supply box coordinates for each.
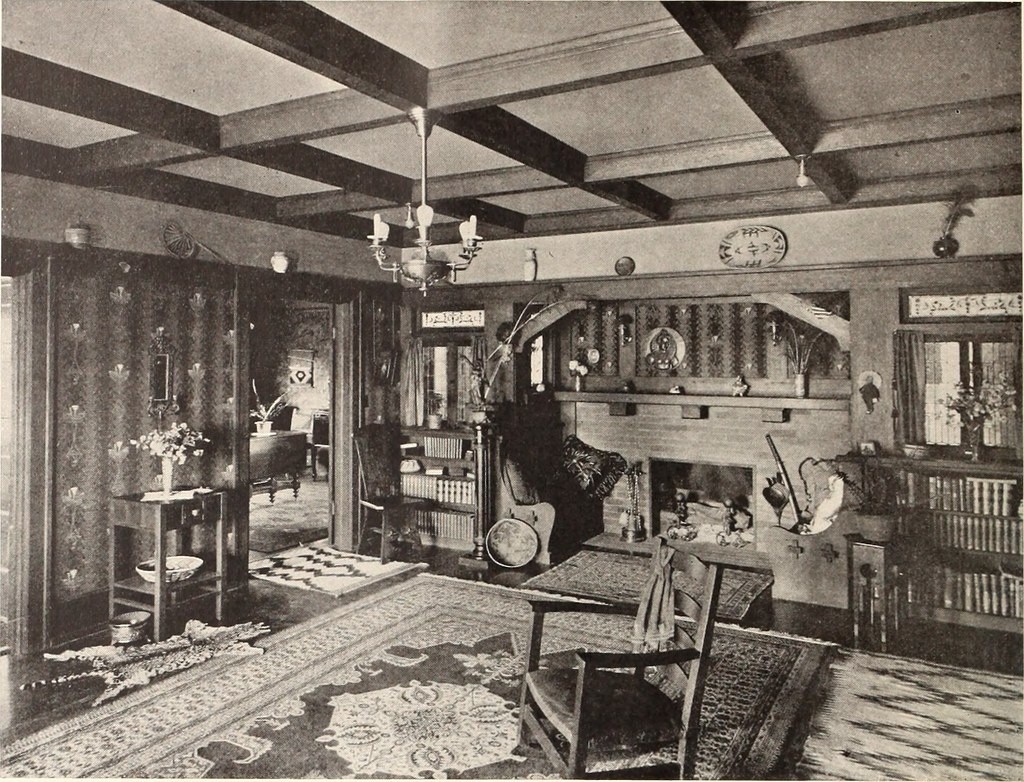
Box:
[350,424,429,565]
[492,399,605,566]
[512,537,726,780]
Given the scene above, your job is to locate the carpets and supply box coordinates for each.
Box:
[514,549,774,621]
[0,570,844,778]
[248,545,420,600]
[250,482,330,553]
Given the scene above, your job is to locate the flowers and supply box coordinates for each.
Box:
[932,355,1022,434]
[128,417,211,466]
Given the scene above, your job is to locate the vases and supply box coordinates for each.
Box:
[965,425,988,464]
[163,458,178,497]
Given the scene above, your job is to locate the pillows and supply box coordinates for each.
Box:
[534,434,629,516]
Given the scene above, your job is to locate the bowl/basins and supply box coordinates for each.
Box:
[109,610,151,644]
[485,518,537,568]
[135,555,204,582]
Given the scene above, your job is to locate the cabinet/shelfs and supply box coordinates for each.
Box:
[398,428,476,519]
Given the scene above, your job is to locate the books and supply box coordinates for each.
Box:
[895,470,1024,619]
[424,437,464,459]
[401,474,476,540]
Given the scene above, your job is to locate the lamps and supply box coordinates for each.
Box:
[366,107,483,296]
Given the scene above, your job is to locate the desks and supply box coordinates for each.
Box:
[249,432,308,503]
[106,485,227,642]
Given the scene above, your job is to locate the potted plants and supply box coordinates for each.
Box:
[423,390,444,431]
[819,438,940,545]
[932,182,979,259]
[248,378,292,438]
[761,293,848,396]
